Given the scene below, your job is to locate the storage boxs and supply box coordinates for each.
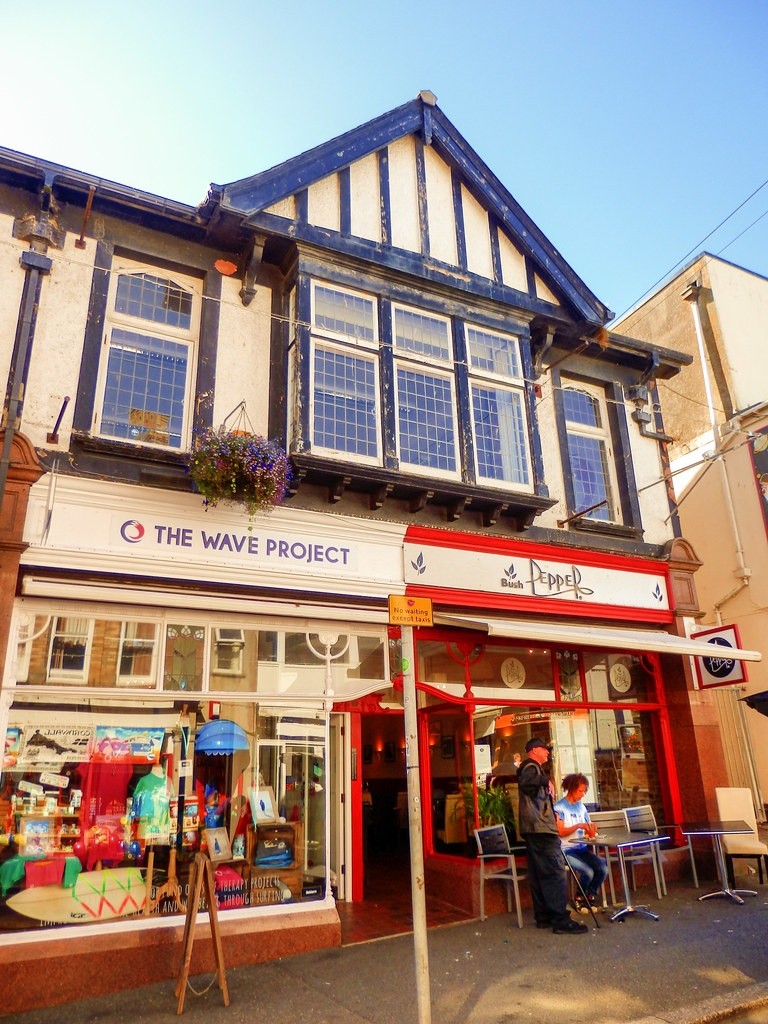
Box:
[211,822,305,905]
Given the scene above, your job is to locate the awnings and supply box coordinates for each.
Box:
[433,612,762,663]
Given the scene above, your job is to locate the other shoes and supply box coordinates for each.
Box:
[537,919,551,928]
[584,894,597,913]
[574,895,589,914]
[554,921,588,933]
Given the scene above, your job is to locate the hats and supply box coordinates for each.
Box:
[525,737,553,753]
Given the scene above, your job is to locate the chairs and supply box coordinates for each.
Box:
[362,782,768,927]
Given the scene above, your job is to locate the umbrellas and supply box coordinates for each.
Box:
[737,690,768,717]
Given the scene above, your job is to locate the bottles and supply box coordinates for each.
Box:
[9,833,14,844]
[59,823,72,835]
[69,823,79,835]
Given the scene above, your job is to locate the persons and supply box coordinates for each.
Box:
[554,772,608,914]
[131,764,177,913]
[516,737,588,934]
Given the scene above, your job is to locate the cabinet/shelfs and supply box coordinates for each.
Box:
[13,805,81,853]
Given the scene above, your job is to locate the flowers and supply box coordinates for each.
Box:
[184,423,294,525]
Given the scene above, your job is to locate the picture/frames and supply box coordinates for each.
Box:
[204,825,233,861]
[440,735,455,760]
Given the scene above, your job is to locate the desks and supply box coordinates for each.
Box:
[682,820,759,904]
[567,832,670,922]
